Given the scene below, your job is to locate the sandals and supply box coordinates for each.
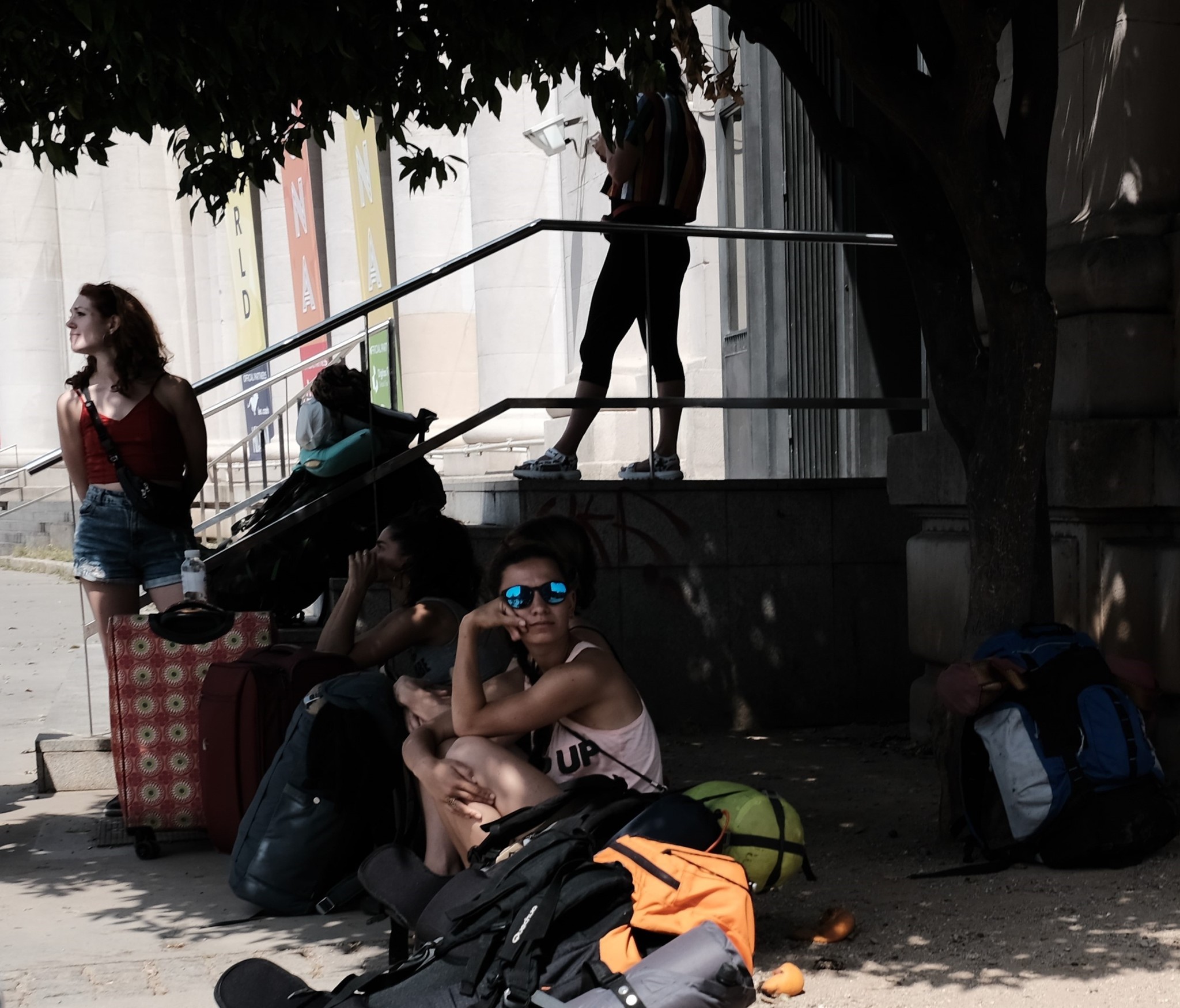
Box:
[620,451,684,480]
[512,449,582,483]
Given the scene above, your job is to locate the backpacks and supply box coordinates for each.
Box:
[334,767,754,1008]
[938,621,1179,871]
[227,667,406,913]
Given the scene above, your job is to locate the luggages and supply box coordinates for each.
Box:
[198,641,360,852]
[107,600,280,861]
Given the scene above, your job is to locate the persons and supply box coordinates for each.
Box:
[54,284,208,816]
[303,513,667,879]
[506,37,705,483]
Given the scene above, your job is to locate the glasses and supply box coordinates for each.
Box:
[498,580,574,607]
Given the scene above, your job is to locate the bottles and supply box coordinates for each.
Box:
[181,550,207,602]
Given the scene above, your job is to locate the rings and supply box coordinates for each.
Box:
[446,796,457,808]
